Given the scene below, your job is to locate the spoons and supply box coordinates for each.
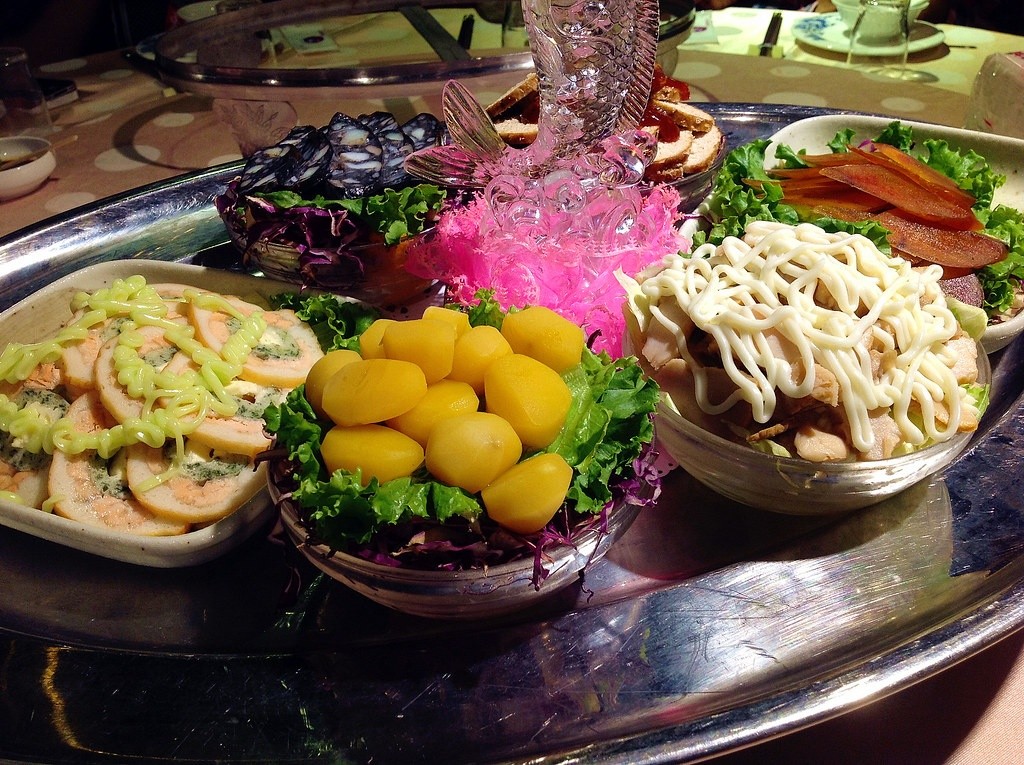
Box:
[758,12,784,60]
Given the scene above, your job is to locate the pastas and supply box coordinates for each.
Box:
[633,218,985,463]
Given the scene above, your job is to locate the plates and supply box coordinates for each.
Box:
[792,11,946,56]
[677,116,1022,356]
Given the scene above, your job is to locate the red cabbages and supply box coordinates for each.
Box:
[258,425,665,590]
[213,103,471,284]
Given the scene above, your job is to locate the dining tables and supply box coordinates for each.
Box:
[0,2,1024,765]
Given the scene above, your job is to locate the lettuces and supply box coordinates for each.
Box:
[688,121,1024,313]
[267,292,658,525]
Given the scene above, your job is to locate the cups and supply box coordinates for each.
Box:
[0,46,54,144]
[500,1,531,52]
[846,0,910,81]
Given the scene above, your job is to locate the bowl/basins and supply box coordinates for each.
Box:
[0,136,57,204]
[831,0,930,40]
[620,319,993,517]
[655,1,696,78]
[178,0,232,23]
[638,132,730,224]
[216,200,442,314]
[0,258,380,566]
[266,415,653,618]
